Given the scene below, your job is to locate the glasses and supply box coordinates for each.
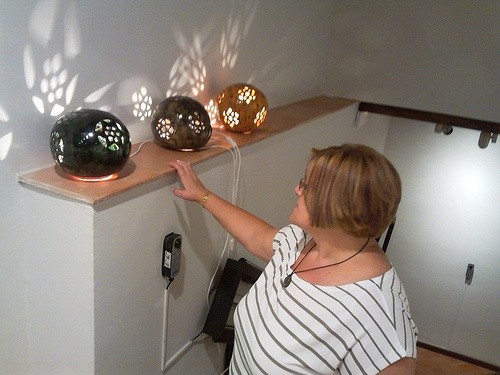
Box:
[297,177,306,191]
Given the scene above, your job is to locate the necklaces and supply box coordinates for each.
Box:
[284,236,369,287]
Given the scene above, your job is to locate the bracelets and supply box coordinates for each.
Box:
[202,192,210,208]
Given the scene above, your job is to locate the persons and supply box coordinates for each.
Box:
[168,143,417,374]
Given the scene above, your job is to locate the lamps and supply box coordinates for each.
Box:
[49,82,269,182]
[478,130,498,149]
